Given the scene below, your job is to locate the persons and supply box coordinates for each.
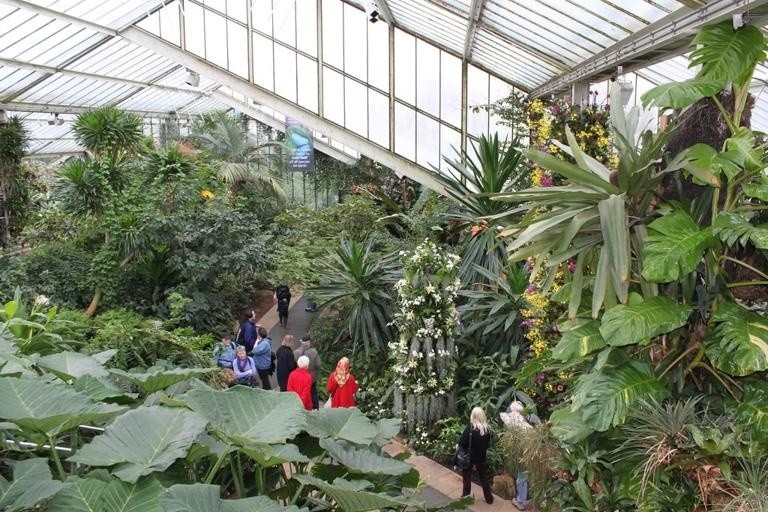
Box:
[276,275,290,329]
[305,266,319,312]
[459,399,547,510]
[213,310,359,409]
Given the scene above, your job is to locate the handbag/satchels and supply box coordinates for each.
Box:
[455,429,472,470]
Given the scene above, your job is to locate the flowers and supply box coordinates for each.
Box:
[518,87,627,421]
[385,234,464,396]
